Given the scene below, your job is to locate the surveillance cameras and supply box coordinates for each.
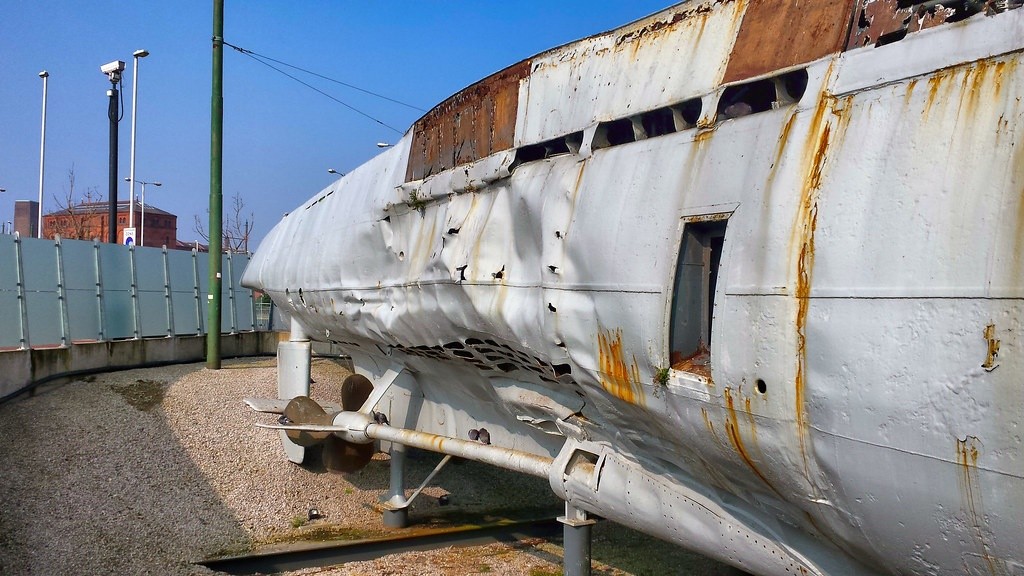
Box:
[101,60,126,74]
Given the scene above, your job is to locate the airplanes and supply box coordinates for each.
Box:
[194,0,1024,576]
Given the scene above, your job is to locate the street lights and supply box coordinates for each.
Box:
[126,176,163,245]
[130,49,149,241]
[36,72,50,240]
